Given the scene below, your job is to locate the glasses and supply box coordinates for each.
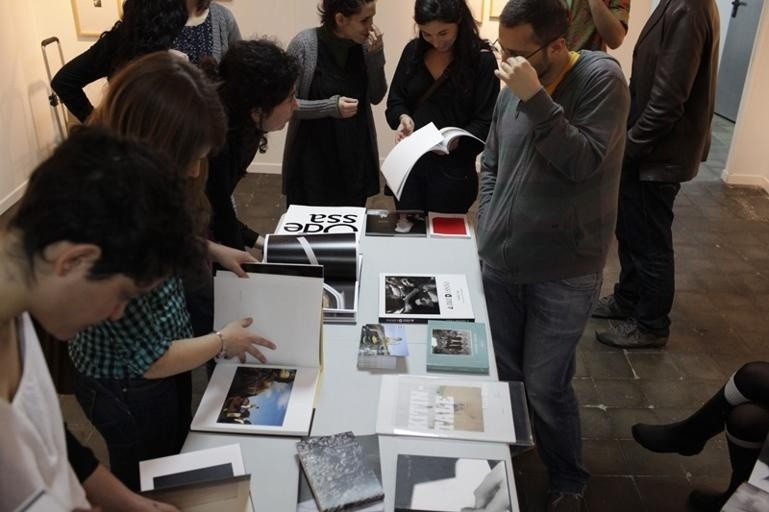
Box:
[489,39,551,60]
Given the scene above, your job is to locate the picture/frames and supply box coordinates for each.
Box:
[488,1,510,19]
[464,0,485,23]
[70,1,123,37]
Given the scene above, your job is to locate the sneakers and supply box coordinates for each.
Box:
[549,490,591,510]
[592,293,629,320]
[595,319,672,350]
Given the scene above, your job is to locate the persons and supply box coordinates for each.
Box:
[385,0,499,217]
[560,0,630,52]
[282,0,387,212]
[475,0,632,512]
[631,361,769,512]
[592,0,720,348]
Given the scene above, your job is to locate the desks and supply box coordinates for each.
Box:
[175,208,521,512]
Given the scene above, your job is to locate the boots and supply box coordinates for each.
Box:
[630,383,736,457]
[687,432,759,511]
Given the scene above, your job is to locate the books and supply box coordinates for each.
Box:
[380,122,486,202]
